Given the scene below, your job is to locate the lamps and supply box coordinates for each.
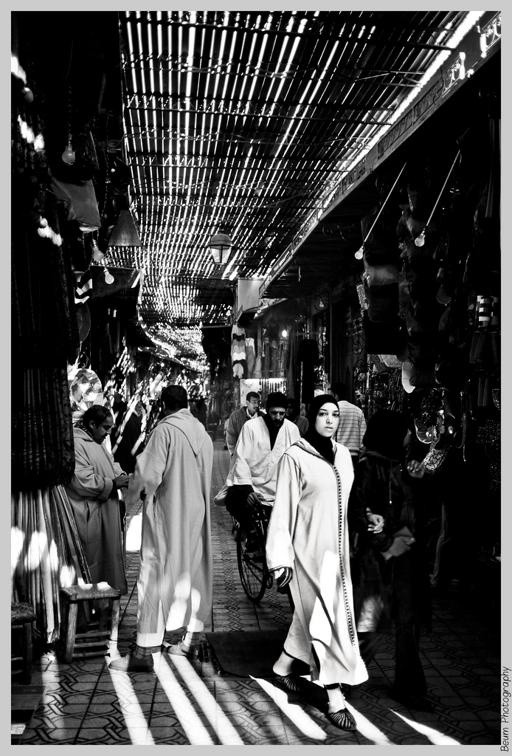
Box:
[352,164,406,261]
[92,239,104,262]
[62,130,75,163]
[208,232,232,267]
[101,258,115,285]
[414,148,461,248]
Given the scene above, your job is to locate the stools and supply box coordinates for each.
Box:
[62,583,122,659]
[11,601,35,685]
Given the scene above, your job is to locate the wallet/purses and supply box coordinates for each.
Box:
[277,567,292,587]
[380,526,416,561]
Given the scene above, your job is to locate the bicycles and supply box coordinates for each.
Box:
[233,508,274,600]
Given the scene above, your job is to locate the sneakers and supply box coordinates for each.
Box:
[109,652,154,672]
[162,639,200,659]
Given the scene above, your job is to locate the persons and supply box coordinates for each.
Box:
[186,386,240,440]
[284,397,309,436]
[63,405,128,614]
[348,408,441,713]
[329,386,366,470]
[226,391,265,462]
[264,394,371,730]
[128,385,213,673]
[106,387,166,474]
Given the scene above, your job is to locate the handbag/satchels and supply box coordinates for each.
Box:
[360,262,408,356]
[402,408,455,477]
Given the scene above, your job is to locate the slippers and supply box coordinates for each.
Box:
[272,665,302,693]
[327,708,357,731]
